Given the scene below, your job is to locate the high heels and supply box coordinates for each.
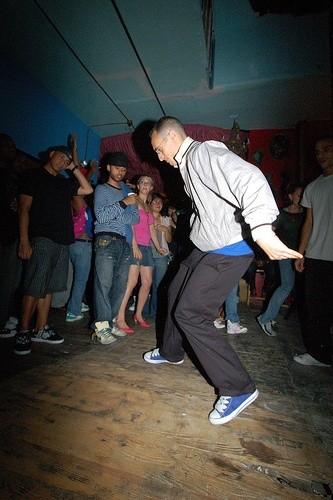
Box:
[116,319,133,333]
[134,314,149,328]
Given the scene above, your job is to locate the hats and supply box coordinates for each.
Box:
[55,146,73,164]
[108,152,128,167]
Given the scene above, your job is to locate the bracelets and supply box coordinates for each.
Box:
[71,165,78,172]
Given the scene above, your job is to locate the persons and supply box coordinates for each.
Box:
[293,133,333,367]
[116,175,167,333]
[92,151,140,346]
[51,256,75,315]
[142,192,171,320]
[11,151,28,175]
[142,116,304,424]
[244,243,281,298]
[213,281,248,335]
[257,181,304,337]
[0,133,23,338]
[12,145,93,355]
[65,160,101,322]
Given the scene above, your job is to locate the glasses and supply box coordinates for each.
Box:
[152,201,163,205]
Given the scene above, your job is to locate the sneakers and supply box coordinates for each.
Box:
[111,326,127,336]
[80,303,90,312]
[95,328,118,345]
[65,312,84,322]
[14,330,31,355]
[208,386,259,425]
[144,347,184,365]
[31,324,64,344]
[293,352,331,367]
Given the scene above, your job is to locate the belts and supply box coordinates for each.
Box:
[75,238,92,242]
[97,232,126,241]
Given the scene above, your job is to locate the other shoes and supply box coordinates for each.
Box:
[9,317,19,324]
[0,328,17,338]
[214,320,226,329]
[227,319,248,334]
[258,316,277,337]
[5,321,16,329]
